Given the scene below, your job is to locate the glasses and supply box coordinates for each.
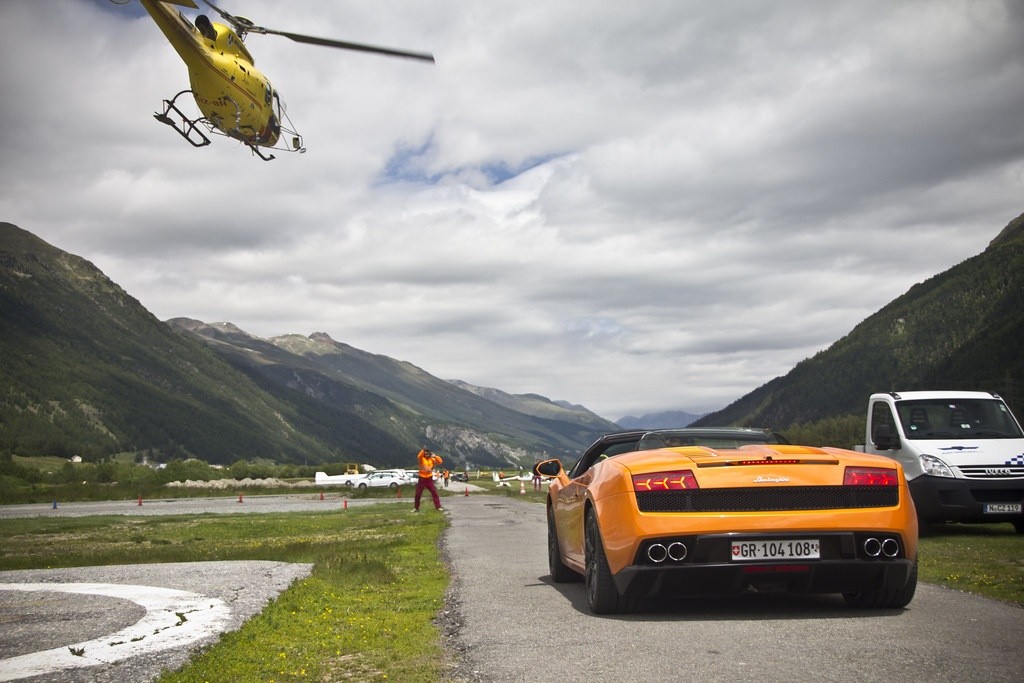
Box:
[424,452,432,455]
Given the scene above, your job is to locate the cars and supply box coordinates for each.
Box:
[314,463,439,490]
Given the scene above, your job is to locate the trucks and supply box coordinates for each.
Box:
[856,390,1024,526]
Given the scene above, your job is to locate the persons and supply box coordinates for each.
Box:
[443,470,449,487]
[533,459,542,492]
[435,469,440,480]
[499,472,504,482]
[464,471,468,478]
[409,450,444,512]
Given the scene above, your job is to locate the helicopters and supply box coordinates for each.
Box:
[138,0,435,167]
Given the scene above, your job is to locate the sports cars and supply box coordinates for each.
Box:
[533,427,923,613]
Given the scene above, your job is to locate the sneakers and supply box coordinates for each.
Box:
[438,508,444,511]
[410,508,418,512]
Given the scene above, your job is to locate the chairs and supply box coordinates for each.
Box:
[637,432,668,451]
[910,408,930,430]
[950,406,968,428]
